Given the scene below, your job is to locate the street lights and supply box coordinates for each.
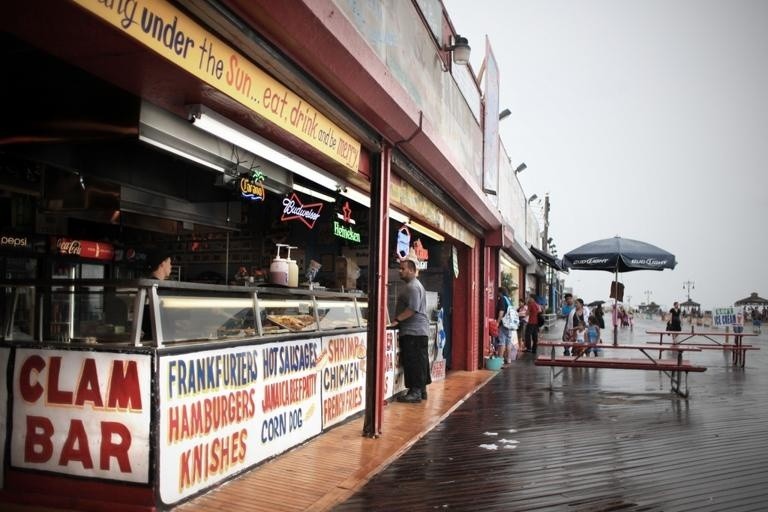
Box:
[643,290,653,310]
[625,295,633,310]
[681,279,696,313]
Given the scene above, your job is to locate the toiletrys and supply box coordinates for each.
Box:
[269,244,301,290]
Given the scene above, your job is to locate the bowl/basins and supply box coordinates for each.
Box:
[485,356,503,370]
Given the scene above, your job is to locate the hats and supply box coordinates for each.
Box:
[144,248,176,269]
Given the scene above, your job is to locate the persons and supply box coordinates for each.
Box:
[136,245,178,340]
[390,259,432,403]
[762,307,766,323]
[751,306,763,334]
[492,286,635,364]
[669,301,684,345]
[765,307,768,323]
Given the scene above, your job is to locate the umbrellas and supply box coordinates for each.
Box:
[561,236,678,345]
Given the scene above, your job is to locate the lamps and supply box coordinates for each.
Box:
[183,103,410,225]
[444,35,471,65]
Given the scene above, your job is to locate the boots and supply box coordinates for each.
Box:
[422,385,427,400]
[397,388,422,403]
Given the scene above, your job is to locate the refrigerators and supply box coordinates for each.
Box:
[33,236,113,353]
[109,245,150,284]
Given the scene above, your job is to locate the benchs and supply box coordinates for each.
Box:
[533,328,759,399]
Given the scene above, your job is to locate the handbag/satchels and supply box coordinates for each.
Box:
[538,311,544,327]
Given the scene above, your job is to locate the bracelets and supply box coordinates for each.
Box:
[395,318,401,323]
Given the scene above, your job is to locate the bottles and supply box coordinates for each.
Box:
[95,242,102,258]
[235,266,248,283]
[51,265,104,342]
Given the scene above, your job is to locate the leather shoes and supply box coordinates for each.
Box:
[522,349,532,352]
[533,350,537,354]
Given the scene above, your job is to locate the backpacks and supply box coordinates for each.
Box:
[500,295,519,330]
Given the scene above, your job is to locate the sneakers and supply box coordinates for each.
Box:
[506,358,511,364]
